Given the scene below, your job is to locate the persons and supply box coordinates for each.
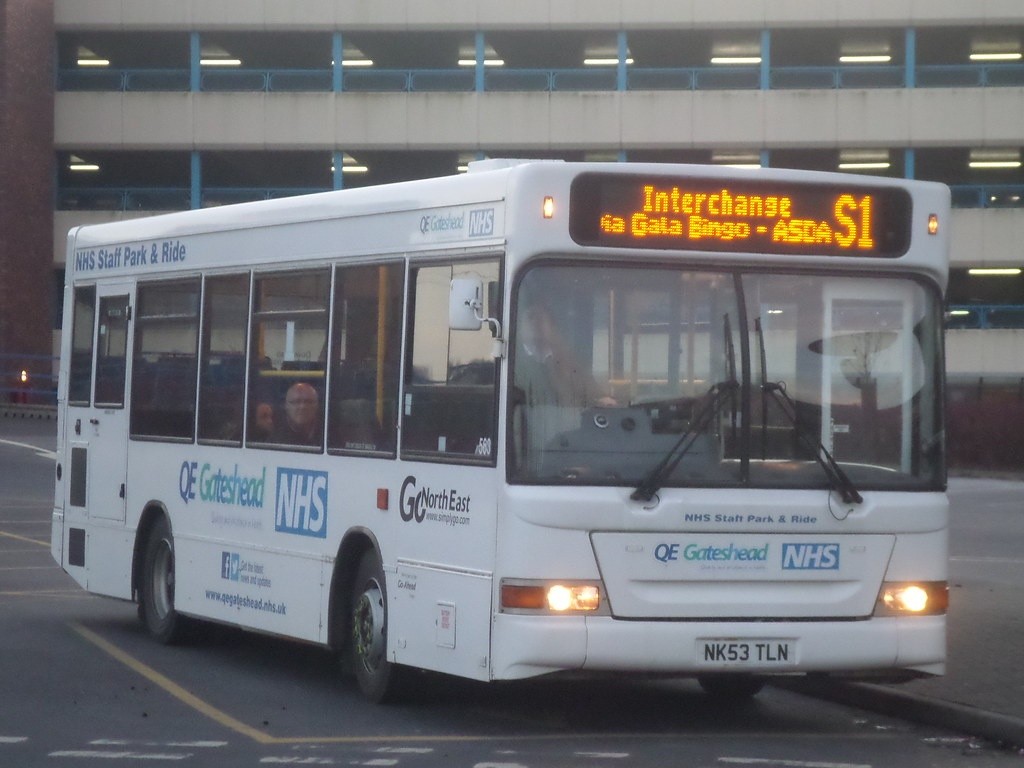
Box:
[267,381,347,449]
[509,305,618,410]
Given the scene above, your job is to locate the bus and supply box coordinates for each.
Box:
[50,158,963,712]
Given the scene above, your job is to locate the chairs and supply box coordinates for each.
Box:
[72,350,494,455]
